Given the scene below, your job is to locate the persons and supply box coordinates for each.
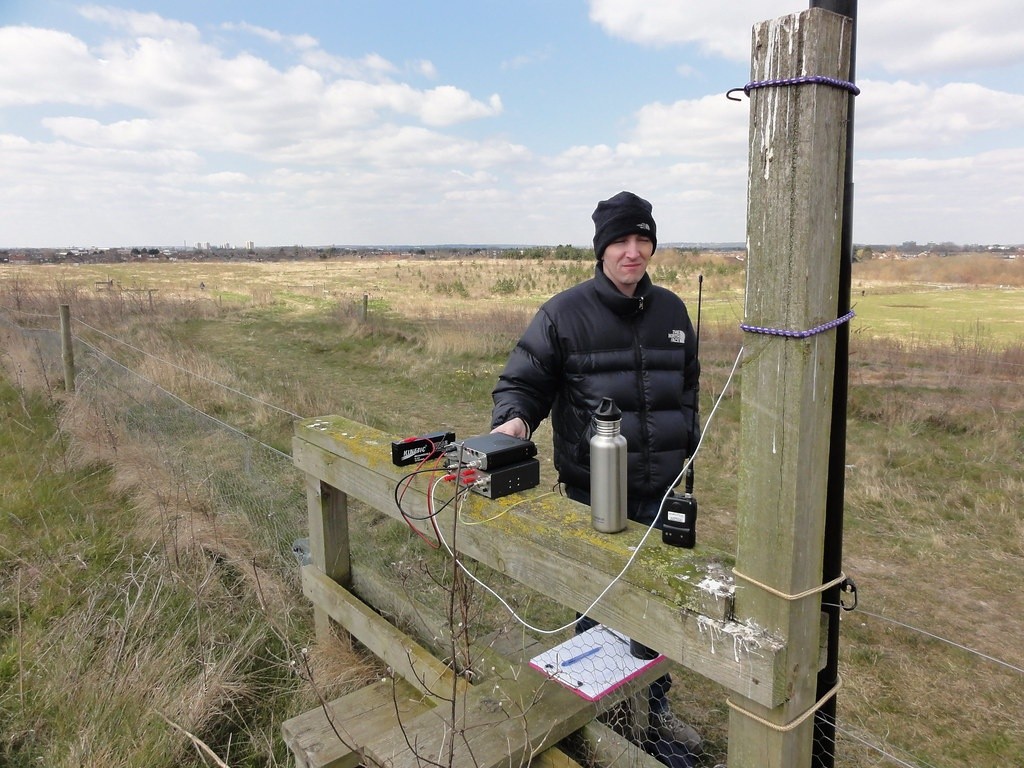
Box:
[490,191,713,760]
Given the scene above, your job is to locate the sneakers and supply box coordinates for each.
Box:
[604,702,632,742]
[648,712,703,754]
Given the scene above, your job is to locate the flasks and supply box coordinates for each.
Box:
[590,397,627,532]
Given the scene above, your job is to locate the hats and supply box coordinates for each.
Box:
[591,191,657,262]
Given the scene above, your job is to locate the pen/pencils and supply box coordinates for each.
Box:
[601,626,630,646]
[561,646,603,666]
